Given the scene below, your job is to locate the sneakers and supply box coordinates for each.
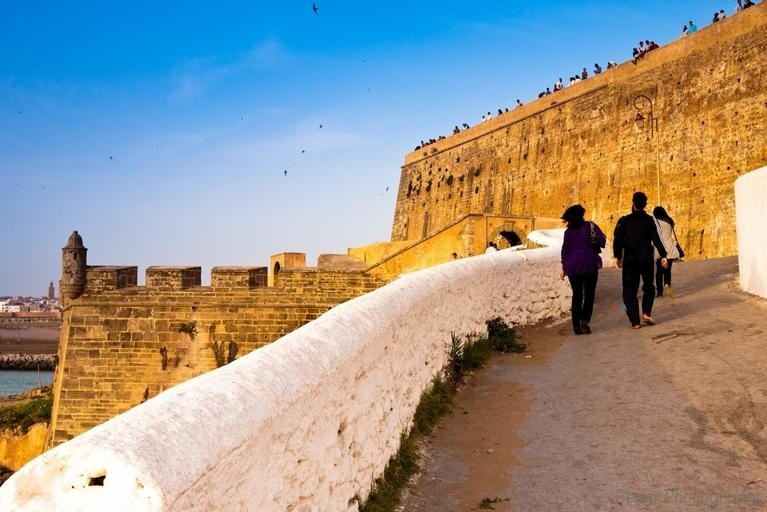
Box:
[642,314,655,325]
[582,321,591,334]
[631,322,640,329]
[657,284,670,297]
[574,328,583,335]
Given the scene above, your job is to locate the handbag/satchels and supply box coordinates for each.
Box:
[590,231,601,247]
[675,244,684,257]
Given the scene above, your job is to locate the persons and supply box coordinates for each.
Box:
[481,99,523,122]
[451,252,457,260]
[631,39,660,65]
[539,61,618,98]
[493,243,500,251]
[652,205,680,298]
[415,135,446,151]
[716,10,726,20]
[712,12,719,22]
[485,241,497,253]
[744,0,755,9]
[558,203,607,335]
[461,123,470,131]
[612,190,670,329]
[680,24,688,38]
[736,0,742,15]
[453,126,460,134]
[687,20,697,33]
